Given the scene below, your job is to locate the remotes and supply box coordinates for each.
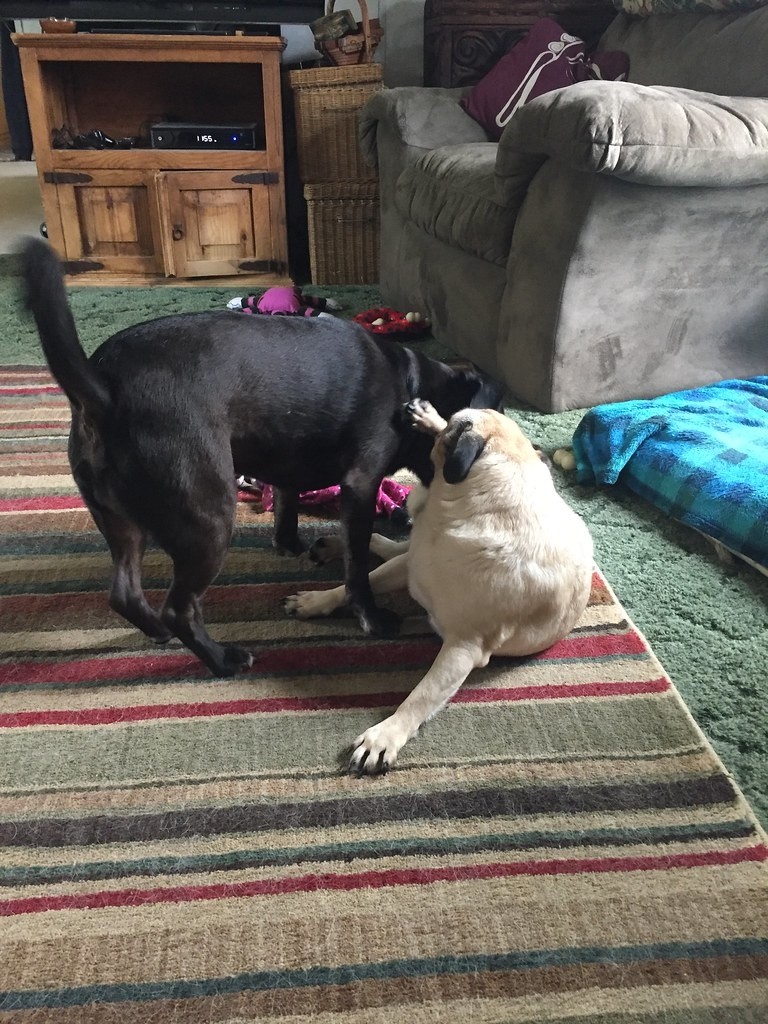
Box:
[118,136,139,150]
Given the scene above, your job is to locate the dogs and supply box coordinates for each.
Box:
[14,238,594,777]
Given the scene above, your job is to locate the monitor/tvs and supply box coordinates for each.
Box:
[0,0,337,36]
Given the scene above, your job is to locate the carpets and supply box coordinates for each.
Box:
[0,366,768,1024]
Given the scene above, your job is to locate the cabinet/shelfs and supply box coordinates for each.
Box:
[11,32,296,288]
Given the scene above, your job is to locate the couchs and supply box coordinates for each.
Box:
[359,0,768,414]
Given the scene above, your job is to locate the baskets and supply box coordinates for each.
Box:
[313,0,384,66]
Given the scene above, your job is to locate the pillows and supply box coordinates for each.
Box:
[458,17,630,141]
